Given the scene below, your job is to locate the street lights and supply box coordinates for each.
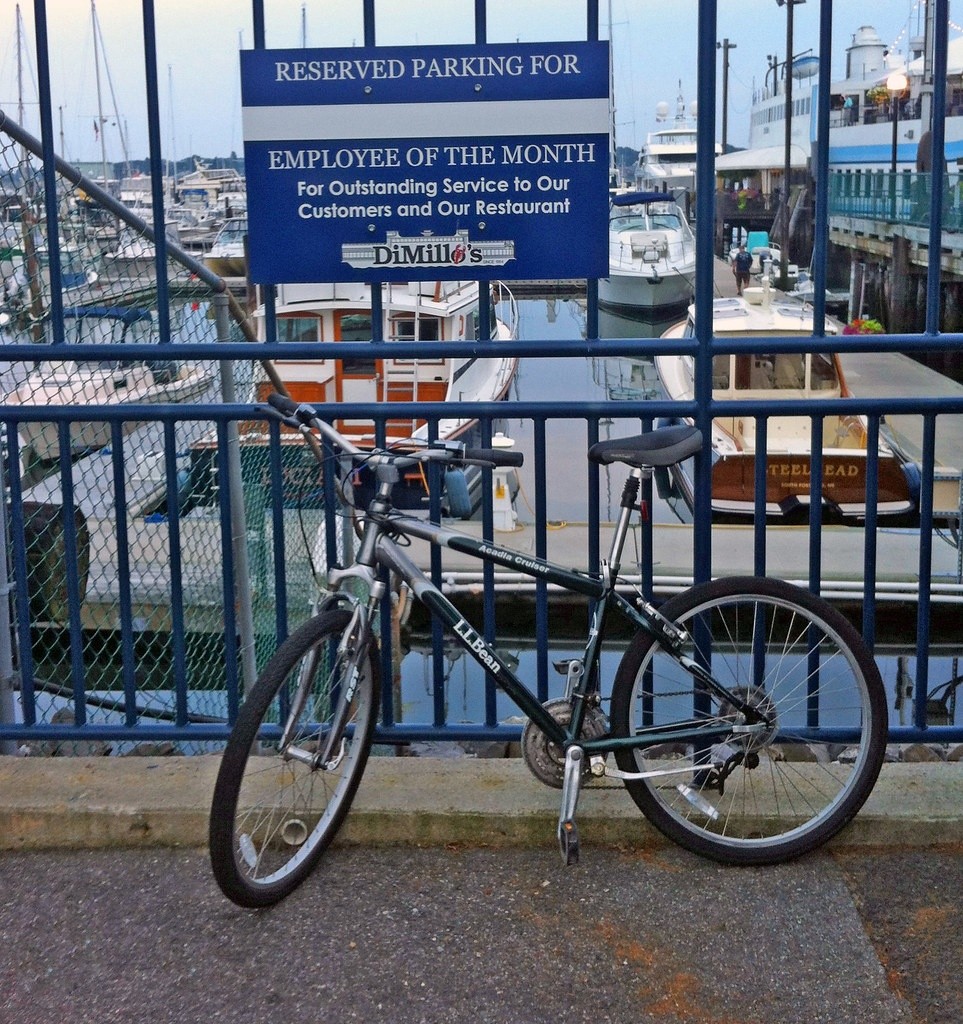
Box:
[775,0,806,278]
[716,38,737,155]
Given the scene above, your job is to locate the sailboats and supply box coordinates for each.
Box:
[0,0,245,318]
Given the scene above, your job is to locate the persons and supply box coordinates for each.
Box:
[843,95,853,125]
[840,96,845,107]
[311,508,421,758]
[732,245,753,296]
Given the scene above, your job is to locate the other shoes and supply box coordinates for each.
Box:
[738,292,741,295]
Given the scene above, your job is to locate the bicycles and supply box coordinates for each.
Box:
[206,393,889,910]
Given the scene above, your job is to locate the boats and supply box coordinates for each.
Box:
[632,1,963,315]
[596,190,695,316]
[652,275,911,526]
[187,280,522,510]
[578,299,690,400]
[0,303,214,462]
